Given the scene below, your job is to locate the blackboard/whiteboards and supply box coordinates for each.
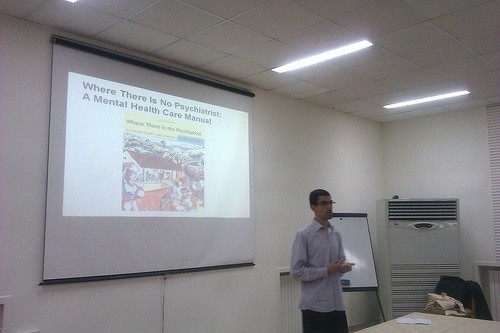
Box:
[327,213,379,293]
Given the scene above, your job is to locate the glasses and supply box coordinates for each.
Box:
[312,200,337,207]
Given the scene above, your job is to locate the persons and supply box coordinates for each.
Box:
[290,189,355,333]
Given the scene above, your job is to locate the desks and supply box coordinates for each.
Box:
[353,311,500,333]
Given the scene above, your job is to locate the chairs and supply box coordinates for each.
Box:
[426,275,493,321]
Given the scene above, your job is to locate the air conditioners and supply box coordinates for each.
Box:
[376,198,461,325]
